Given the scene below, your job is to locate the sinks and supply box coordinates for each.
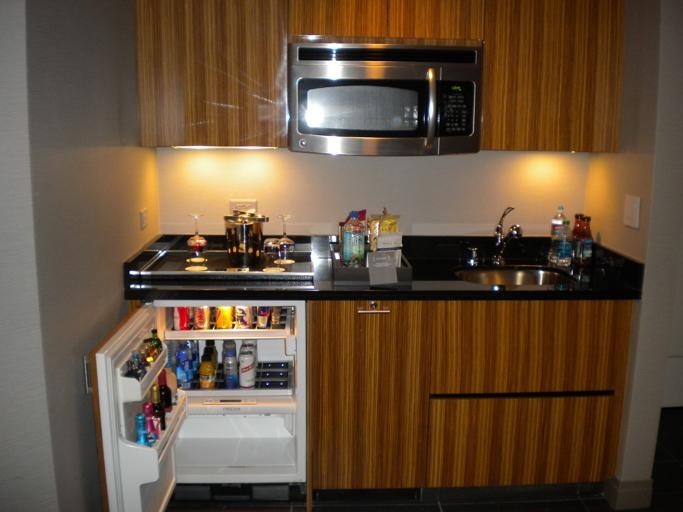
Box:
[456,264,579,290]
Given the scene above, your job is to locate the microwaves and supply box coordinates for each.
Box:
[287,33,483,156]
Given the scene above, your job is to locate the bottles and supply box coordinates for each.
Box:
[344,212,365,267]
[122,306,283,448]
[547,205,594,268]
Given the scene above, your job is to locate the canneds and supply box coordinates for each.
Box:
[172,306,253,330]
[237,339,257,388]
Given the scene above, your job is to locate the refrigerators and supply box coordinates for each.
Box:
[97,298,308,511]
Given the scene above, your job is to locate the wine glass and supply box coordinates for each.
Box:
[275,214,296,264]
[187,212,208,262]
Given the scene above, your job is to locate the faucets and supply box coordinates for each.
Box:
[495,206,522,265]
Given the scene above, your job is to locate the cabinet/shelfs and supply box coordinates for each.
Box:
[430,301,635,488]
[306,300,425,502]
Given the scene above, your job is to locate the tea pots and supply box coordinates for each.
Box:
[222,209,270,267]
